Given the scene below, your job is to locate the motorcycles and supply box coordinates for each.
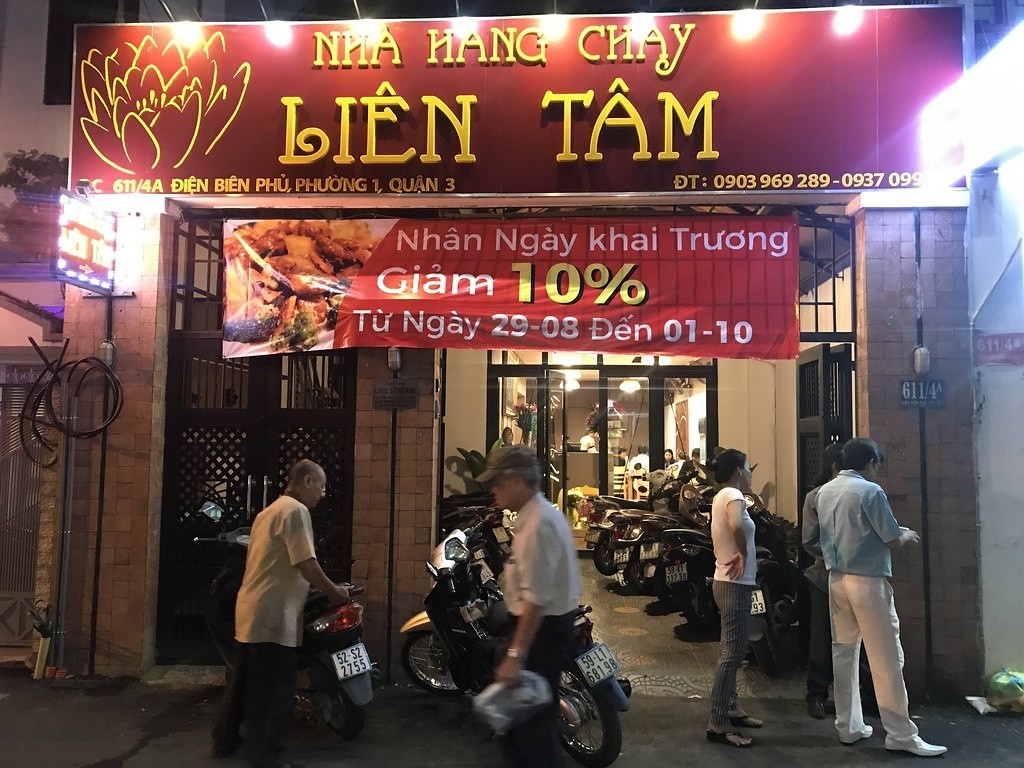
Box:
[396,513,633,768]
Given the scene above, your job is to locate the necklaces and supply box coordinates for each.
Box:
[283,493,309,507]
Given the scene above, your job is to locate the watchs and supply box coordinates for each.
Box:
[506,648,527,658]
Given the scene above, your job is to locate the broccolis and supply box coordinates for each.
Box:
[225,287,347,354]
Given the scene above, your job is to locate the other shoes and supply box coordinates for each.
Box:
[213,736,242,758]
[807,694,825,719]
[254,751,305,768]
[823,696,836,713]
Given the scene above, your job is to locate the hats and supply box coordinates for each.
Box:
[475,444,536,483]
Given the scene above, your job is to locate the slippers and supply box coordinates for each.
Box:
[729,712,761,727]
[706,726,751,746]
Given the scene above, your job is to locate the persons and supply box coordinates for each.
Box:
[567,435,580,452]
[664,450,675,468]
[628,444,649,472]
[212,457,355,768]
[617,447,628,466]
[489,427,514,450]
[814,437,948,756]
[475,445,584,768]
[707,449,762,749]
[678,449,700,479]
[801,444,879,719]
[679,451,690,462]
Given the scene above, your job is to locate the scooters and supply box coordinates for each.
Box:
[438,448,810,681]
[192,500,377,743]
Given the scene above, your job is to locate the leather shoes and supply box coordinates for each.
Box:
[861,725,873,739]
[885,735,948,756]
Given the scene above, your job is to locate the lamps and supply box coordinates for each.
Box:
[386,346,402,372]
[98,338,118,370]
[909,343,931,376]
